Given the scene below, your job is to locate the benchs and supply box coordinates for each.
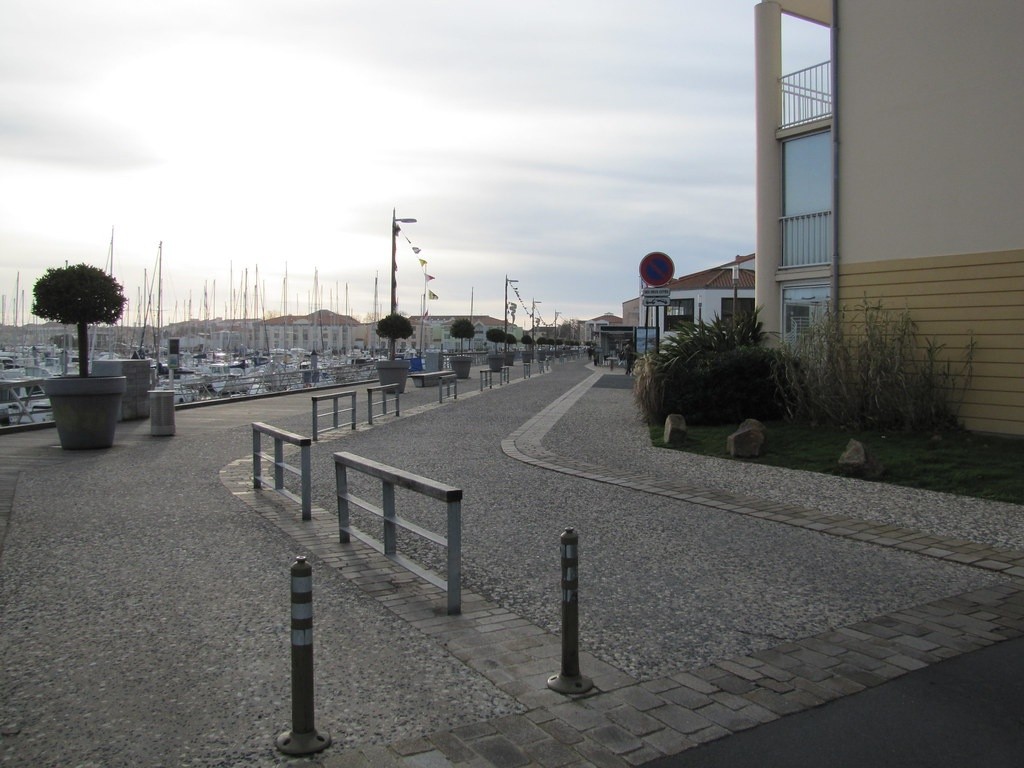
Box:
[408,370,455,388]
[607,357,619,371]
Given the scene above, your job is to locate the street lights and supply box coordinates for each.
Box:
[504,274,519,354]
[532,298,542,362]
[554,309,561,356]
[389,207,417,359]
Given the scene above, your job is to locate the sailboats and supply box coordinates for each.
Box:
[0,225,420,424]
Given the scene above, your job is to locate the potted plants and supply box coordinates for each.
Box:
[536,337,547,361]
[486,329,506,373]
[376,315,413,394]
[450,319,474,378]
[521,334,533,364]
[30,263,127,452]
[504,334,516,365]
[546,339,555,359]
[556,339,562,357]
